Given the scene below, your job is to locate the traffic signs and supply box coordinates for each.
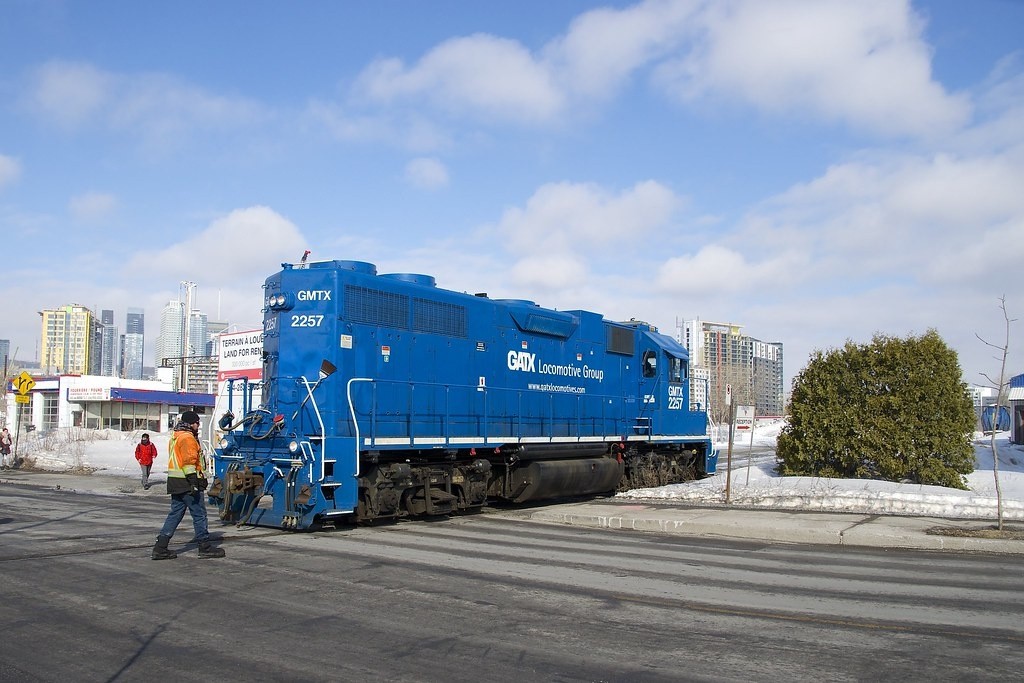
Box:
[734,404,756,433]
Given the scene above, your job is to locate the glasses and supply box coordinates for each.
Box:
[196,421,199,425]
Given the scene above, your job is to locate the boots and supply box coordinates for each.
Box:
[197,539,225,559]
[149,535,177,560]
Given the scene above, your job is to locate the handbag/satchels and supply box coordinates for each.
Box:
[1,448,7,455]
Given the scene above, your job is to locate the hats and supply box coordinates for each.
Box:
[181,411,199,424]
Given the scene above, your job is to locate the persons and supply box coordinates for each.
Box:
[135,433,157,489]
[151,411,225,560]
[0,428,12,471]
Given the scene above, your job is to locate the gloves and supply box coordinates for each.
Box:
[187,484,199,497]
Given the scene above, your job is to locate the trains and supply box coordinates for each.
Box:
[207,249,724,530]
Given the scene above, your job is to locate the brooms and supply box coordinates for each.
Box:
[292,359,337,421]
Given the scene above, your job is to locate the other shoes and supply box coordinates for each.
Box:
[5,465,10,471]
[143,484,149,490]
[1,467,6,472]
